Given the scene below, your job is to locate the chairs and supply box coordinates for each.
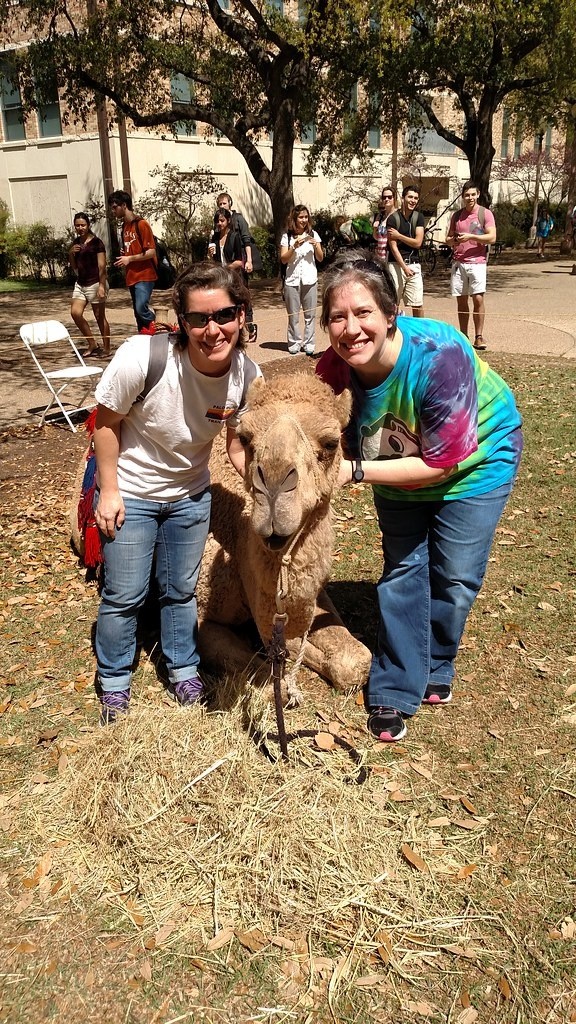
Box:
[19,319,104,433]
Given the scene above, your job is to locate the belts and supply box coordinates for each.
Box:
[388,258,419,264]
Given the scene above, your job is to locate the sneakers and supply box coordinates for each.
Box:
[422,682,453,704]
[166,677,216,710]
[101,689,130,724]
[367,705,407,741]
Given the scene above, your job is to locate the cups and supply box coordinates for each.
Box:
[209,243,217,254]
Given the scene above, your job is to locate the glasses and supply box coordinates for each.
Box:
[382,196,392,200]
[378,207,386,210]
[182,304,239,329]
[111,203,120,210]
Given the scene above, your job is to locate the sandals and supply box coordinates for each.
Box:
[472,335,486,348]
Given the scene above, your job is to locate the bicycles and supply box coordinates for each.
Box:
[417,228,455,276]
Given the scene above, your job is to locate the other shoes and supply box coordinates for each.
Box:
[537,253,545,259]
[304,346,314,355]
[288,346,299,353]
[569,264,576,275]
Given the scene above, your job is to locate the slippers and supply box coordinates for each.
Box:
[82,343,100,356]
[96,352,112,358]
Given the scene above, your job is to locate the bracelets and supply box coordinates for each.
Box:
[350,461,353,481]
[293,246,295,249]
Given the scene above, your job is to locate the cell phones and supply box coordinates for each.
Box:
[301,236,311,242]
[452,231,460,241]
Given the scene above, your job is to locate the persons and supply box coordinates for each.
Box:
[280,204,323,356]
[370,186,398,262]
[386,185,425,318]
[315,248,524,741]
[209,208,242,270]
[107,190,158,335]
[210,193,257,343]
[68,212,112,357]
[92,260,266,726]
[445,181,496,348]
[369,202,385,223]
[534,209,553,259]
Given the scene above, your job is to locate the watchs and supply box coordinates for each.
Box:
[353,458,364,483]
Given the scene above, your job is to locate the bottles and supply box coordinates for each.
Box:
[163,256,169,267]
[381,226,386,235]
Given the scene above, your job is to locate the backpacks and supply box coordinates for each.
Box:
[121,218,176,290]
